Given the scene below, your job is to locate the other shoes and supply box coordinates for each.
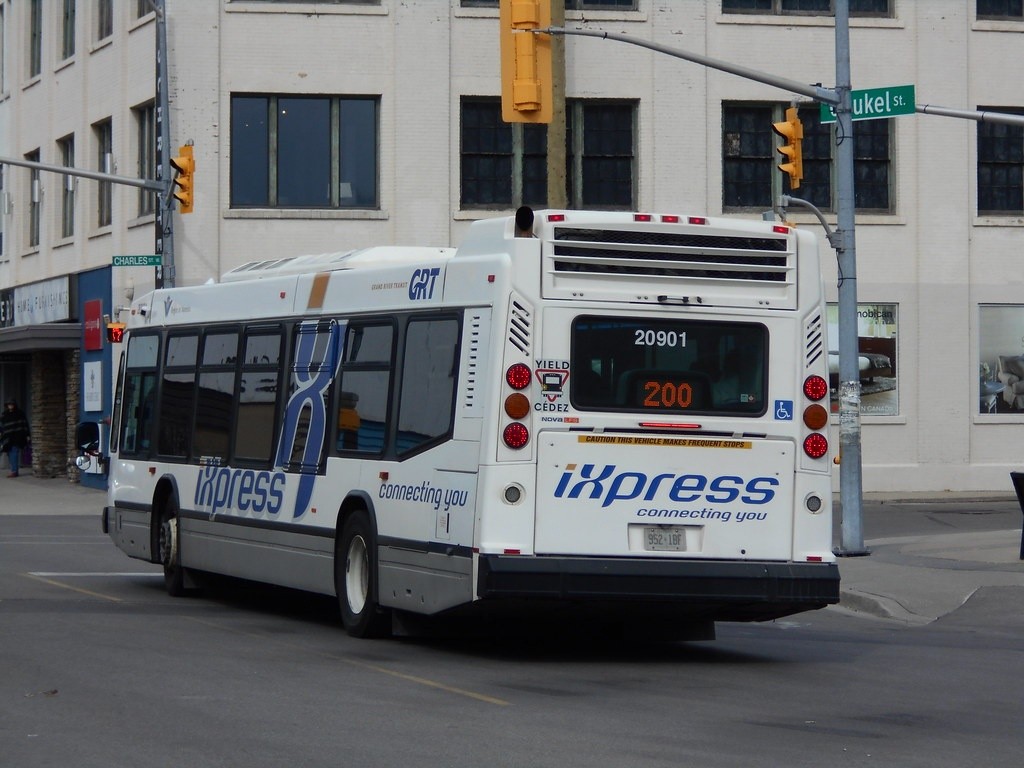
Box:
[5,471,19,477]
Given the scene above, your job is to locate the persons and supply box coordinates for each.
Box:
[1,398,31,478]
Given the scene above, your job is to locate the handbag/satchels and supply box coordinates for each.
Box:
[21,439,32,466]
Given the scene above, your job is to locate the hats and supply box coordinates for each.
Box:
[4,396,18,406]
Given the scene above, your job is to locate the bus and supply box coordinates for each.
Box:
[75,205,842,638]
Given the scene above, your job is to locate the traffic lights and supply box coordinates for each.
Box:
[168,146,195,213]
[774,108,803,189]
